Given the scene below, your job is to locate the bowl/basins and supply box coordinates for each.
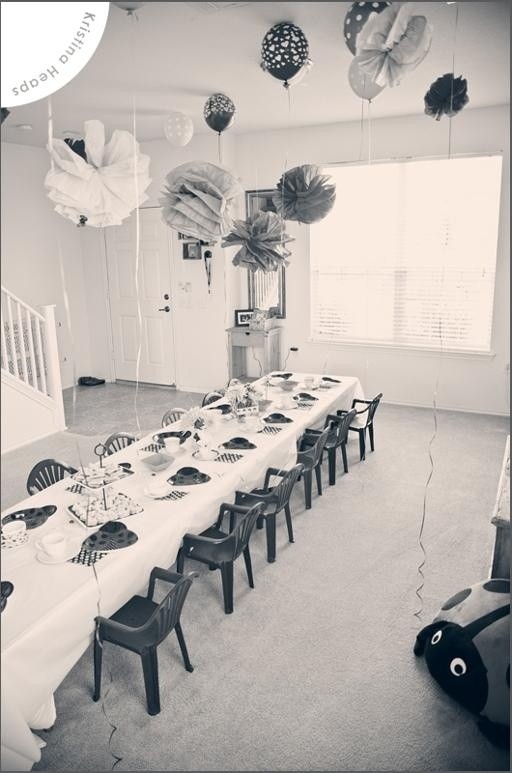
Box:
[96,521,128,543]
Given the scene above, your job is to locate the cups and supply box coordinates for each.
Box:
[3,520,27,542]
[41,533,65,554]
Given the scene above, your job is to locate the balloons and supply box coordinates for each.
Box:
[349,56,386,98]
[111,1,152,8]
[163,111,195,146]
[204,92,236,129]
[343,1,390,53]
[260,22,306,79]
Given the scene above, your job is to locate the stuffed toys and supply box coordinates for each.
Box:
[415,577,509,752]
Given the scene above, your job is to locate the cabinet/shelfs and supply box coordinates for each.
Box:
[489,434,511,580]
[225,325,282,386]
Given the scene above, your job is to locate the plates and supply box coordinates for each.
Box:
[2,505,58,529]
[69,462,136,490]
[80,521,138,549]
[37,543,83,565]
[143,372,338,499]
[1,535,39,548]
[1,580,14,613]
[67,492,145,529]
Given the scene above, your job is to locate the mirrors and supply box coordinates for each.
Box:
[245,190,287,319]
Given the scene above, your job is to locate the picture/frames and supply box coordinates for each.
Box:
[235,309,254,328]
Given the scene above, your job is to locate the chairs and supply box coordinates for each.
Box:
[339,392,383,461]
[103,431,140,460]
[296,426,330,511]
[26,457,80,498]
[235,464,306,563]
[92,566,193,715]
[203,391,222,409]
[304,409,357,486]
[176,503,266,614]
[162,408,188,428]
